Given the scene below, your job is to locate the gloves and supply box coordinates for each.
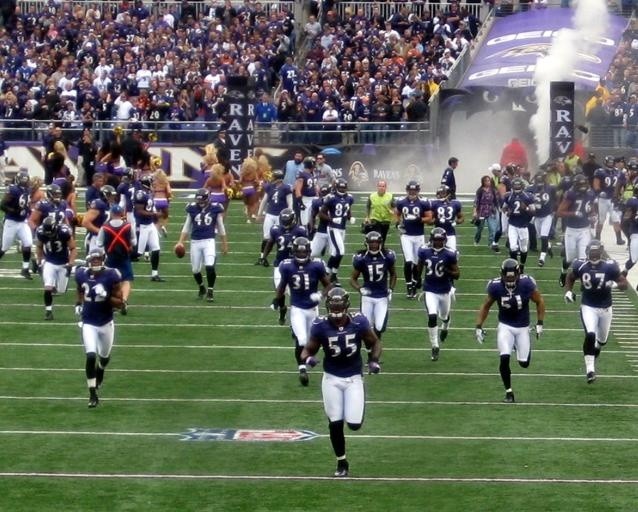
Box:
[475,327,485,344]
[304,356,321,367]
[368,358,380,374]
[535,324,543,340]
[564,291,575,303]
[75,304,82,315]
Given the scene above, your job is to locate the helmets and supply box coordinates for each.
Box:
[326,287,349,317]
[196,189,210,208]
[585,239,603,265]
[506,153,638,194]
[42,216,58,237]
[15,171,153,214]
[270,168,451,202]
[428,227,446,252]
[500,259,520,281]
[292,237,311,264]
[87,249,107,272]
[364,231,383,255]
[279,208,297,229]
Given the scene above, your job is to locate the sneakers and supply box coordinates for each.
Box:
[199,284,214,301]
[559,273,567,287]
[279,306,287,325]
[21,269,32,280]
[121,300,128,315]
[87,365,104,407]
[45,310,53,320]
[334,459,348,476]
[431,329,447,361]
[151,276,164,281]
[587,372,595,384]
[299,373,308,386]
[504,391,514,403]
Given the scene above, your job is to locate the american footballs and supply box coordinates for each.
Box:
[175,245,185,258]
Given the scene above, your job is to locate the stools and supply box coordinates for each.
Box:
[141,115,206,141]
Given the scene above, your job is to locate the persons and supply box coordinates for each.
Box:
[348,231,399,368]
[474,258,546,405]
[409,226,462,362]
[73,244,125,408]
[300,287,382,478]
[563,241,628,384]
[0,1,638,325]
[269,236,333,387]
